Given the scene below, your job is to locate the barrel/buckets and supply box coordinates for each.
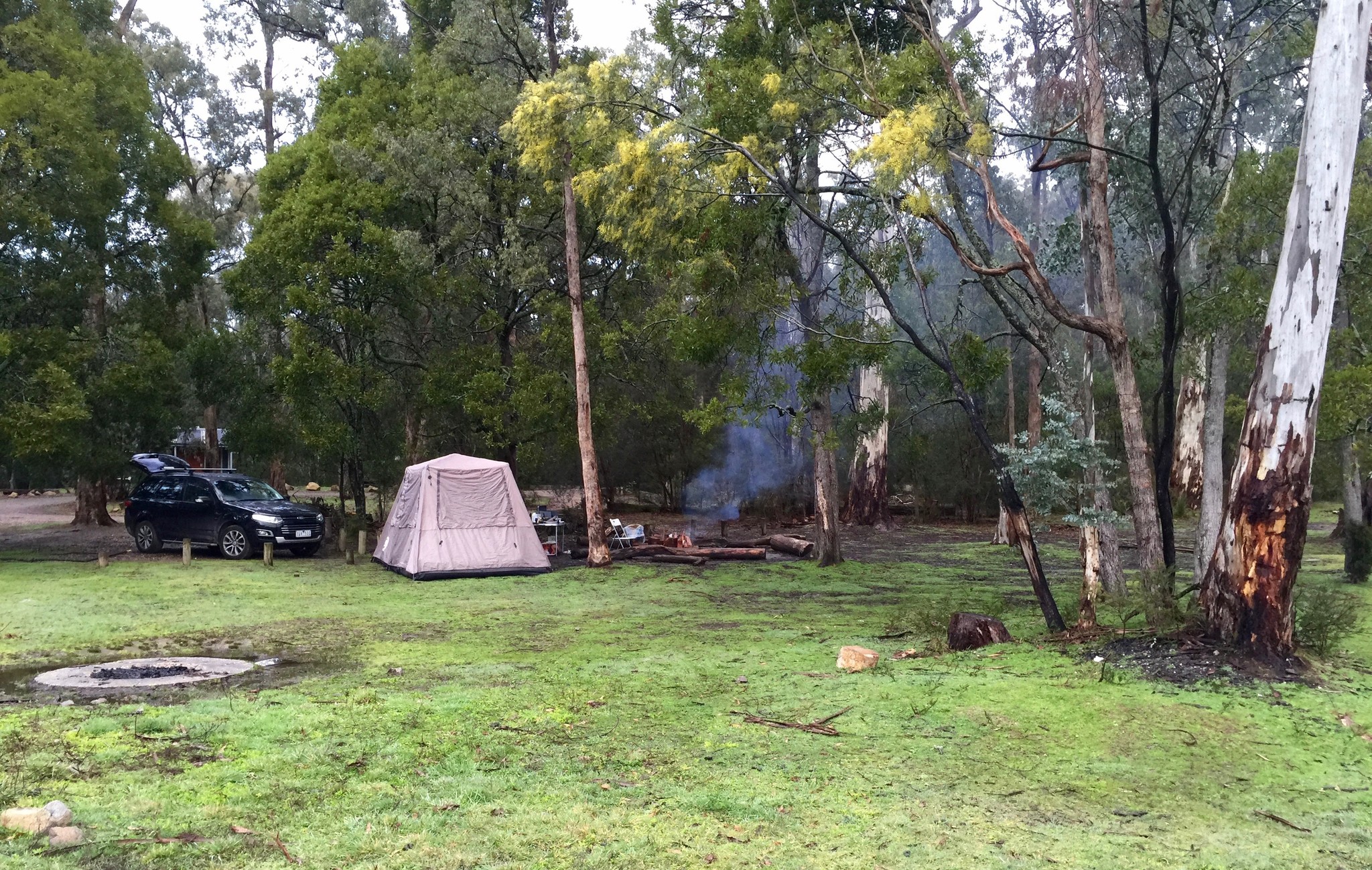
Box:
[625,528,642,546]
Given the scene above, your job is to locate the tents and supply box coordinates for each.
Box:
[370,453,550,580]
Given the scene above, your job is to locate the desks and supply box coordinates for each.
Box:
[533,521,566,557]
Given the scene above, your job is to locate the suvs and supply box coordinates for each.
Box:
[119,452,326,559]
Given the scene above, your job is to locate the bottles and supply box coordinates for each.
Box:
[535,506,540,522]
[542,513,545,521]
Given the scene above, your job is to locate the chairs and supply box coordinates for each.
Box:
[608,518,638,551]
